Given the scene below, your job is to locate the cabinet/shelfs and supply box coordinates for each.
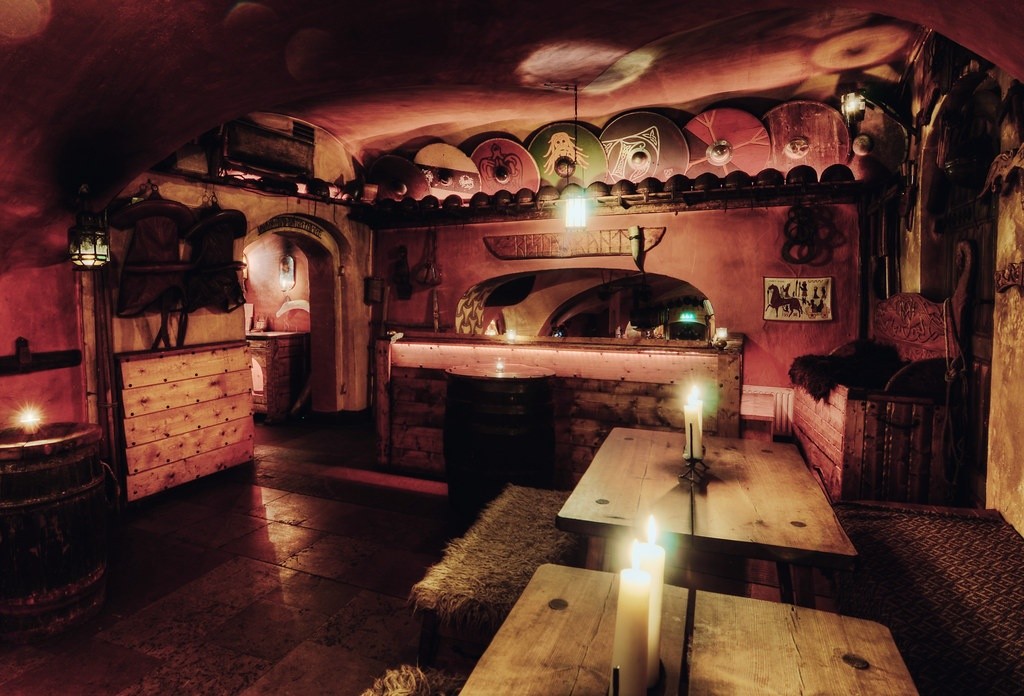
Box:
[247,331,308,411]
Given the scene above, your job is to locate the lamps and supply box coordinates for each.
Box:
[70,208,112,271]
[840,88,866,123]
[564,84,587,231]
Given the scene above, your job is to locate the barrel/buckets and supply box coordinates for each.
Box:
[443,364,557,529]
[0,422,110,647]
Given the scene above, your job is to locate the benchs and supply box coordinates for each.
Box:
[411,485,584,665]
[791,241,971,501]
[789,497,1024,696]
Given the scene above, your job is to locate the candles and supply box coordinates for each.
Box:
[683,385,704,458]
[19,406,39,434]
[608,538,652,695]
[632,512,666,686]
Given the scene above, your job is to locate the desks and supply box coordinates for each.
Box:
[458,564,919,696]
[445,363,556,486]
[556,422,856,603]
[0,421,110,640]
